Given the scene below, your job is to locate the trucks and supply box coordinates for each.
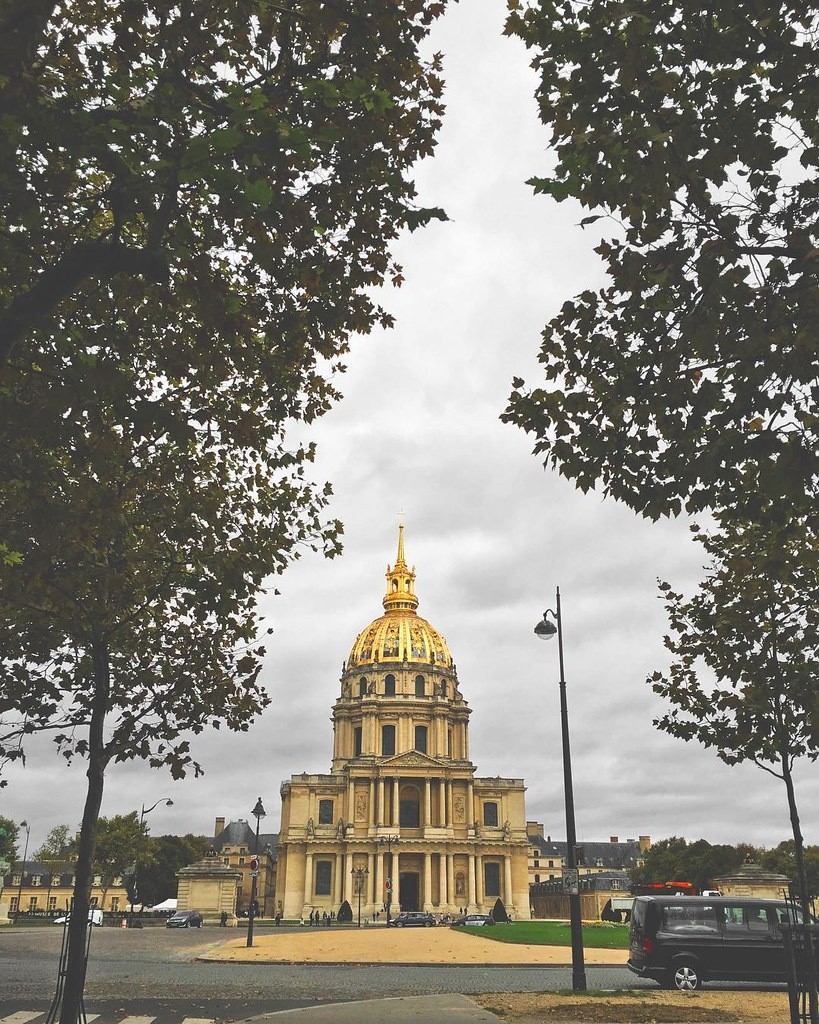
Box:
[53,909,103,928]
[608,890,723,918]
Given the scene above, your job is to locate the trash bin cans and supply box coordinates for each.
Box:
[121,918,127,929]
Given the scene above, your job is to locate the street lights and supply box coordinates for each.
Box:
[127,796,174,920]
[379,834,402,913]
[243,796,268,947]
[350,864,370,927]
[12,819,31,924]
[536,585,595,991]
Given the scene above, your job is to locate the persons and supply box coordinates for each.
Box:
[307,817,314,835]
[460,906,468,916]
[504,819,511,838]
[275,915,280,927]
[309,910,335,927]
[337,818,344,835]
[243,909,264,919]
[432,912,451,921]
[218,911,227,927]
[474,820,481,835]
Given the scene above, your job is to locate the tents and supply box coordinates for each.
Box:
[153,899,178,912]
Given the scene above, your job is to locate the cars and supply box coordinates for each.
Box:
[452,914,495,926]
[166,909,203,929]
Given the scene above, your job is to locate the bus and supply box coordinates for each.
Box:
[632,881,697,900]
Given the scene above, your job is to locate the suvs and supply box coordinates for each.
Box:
[388,912,436,927]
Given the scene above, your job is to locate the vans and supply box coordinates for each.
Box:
[626,897,819,991]
